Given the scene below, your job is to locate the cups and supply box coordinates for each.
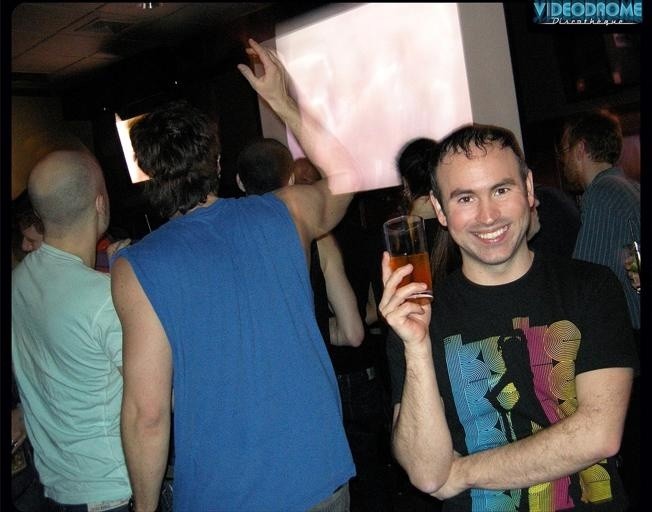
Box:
[383,215,435,306]
[623,242,641,294]
